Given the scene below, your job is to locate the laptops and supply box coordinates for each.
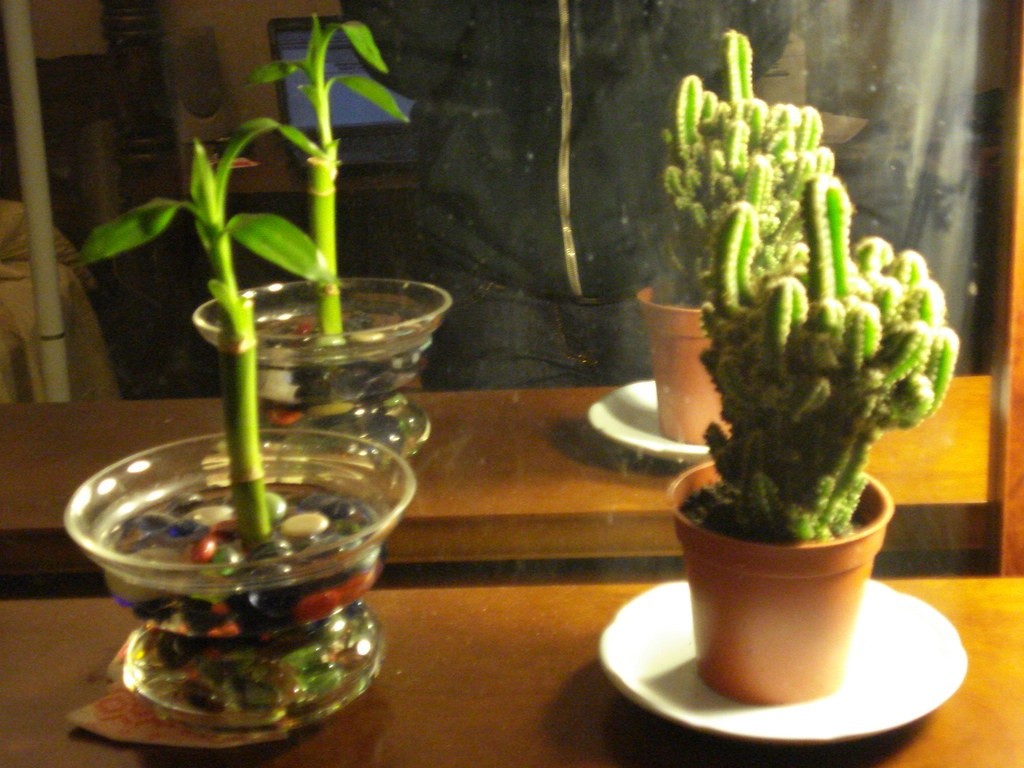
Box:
[269,16,419,172]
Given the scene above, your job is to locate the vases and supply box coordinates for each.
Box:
[63,424,420,733]
[189,274,453,461]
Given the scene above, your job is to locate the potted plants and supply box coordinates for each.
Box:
[667,155,961,706]
[635,28,836,446]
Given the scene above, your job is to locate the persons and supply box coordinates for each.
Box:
[340,0,794,392]
